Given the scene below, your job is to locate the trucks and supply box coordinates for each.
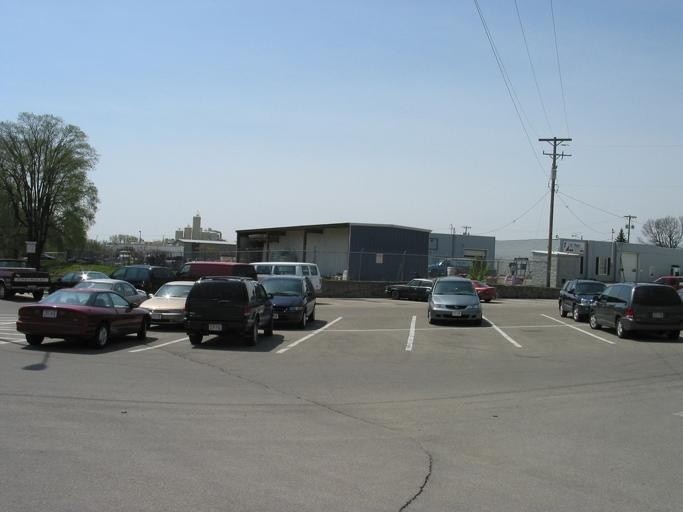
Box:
[113,247,135,266]
[430,258,476,277]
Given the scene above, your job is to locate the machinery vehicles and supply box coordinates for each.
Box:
[503,256,528,285]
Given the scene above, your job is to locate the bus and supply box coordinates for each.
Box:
[248,262,322,298]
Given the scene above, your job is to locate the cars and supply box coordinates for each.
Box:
[15,288,151,350]
[71,278,152,308]
[137,280,196,326]
[384,278,433,302]
[471,279,496,302]
[675,282,682,303]
[47,268,111,295]
[40,253,76,265]
[257,275,316,329]
[425,277,483,325]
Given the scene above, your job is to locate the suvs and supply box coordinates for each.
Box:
[183,276,274,346]
[558,279,607,322]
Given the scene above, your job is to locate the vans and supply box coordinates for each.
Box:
[108,265,170,299]
[651,275,683,290]
[174,261,258,282]
[588,281,683,339]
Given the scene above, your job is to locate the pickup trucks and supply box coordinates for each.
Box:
[0,259,49,300]
